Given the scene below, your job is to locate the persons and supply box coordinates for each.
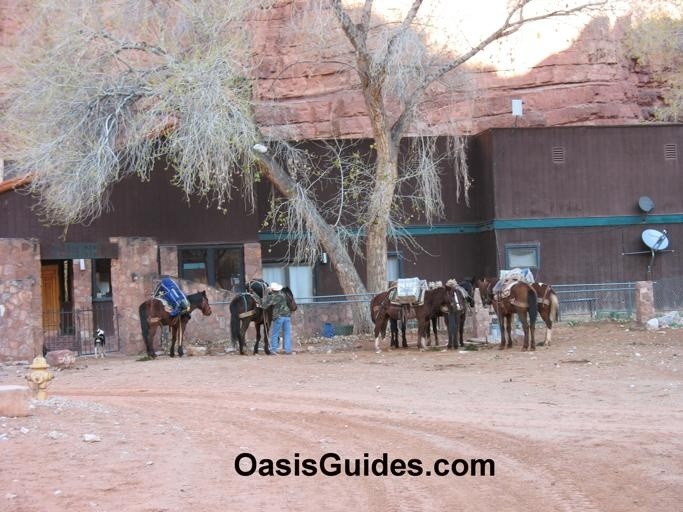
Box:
[260,282,293,354]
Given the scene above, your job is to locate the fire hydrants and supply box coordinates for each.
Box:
[25,353,54,401]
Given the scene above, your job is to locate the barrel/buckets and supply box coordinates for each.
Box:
[324,322,332,336]
[489,323,501,339]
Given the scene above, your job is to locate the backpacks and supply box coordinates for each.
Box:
[247,278,269,298]
[154,278,191,313]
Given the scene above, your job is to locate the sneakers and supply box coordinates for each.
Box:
[281,349,292,354]
[270,349,276,355]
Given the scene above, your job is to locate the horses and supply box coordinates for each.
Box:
[443,278,476,350]
[370,283,463,354]
[478,278,538,353]
[229,287,298,357]
[389,279,441,348]
[496,278,559,347]
[139,288,212,360]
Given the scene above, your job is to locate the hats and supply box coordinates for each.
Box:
[267,282,283,291]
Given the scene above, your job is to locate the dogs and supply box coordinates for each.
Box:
[94,328,106,360]
